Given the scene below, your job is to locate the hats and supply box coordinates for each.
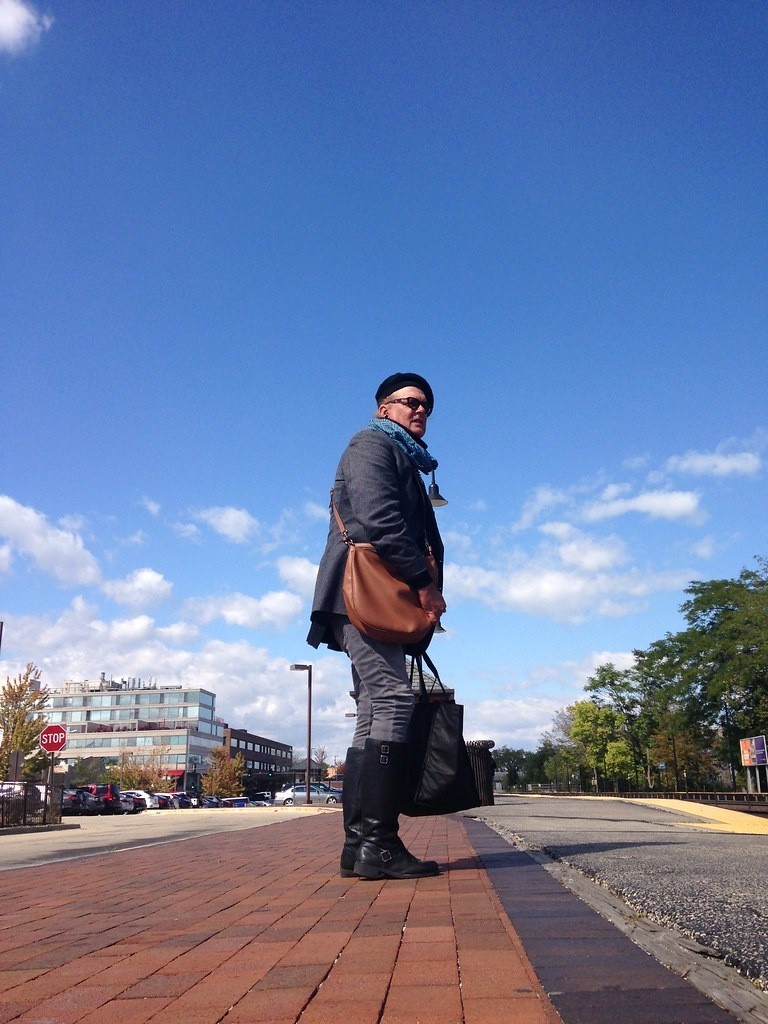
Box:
[375,372,434,417]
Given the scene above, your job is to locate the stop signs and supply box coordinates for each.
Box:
[39,724,67,752]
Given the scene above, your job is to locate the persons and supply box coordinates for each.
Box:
[331,374,446,879]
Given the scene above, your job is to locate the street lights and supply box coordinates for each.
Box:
[704,700,737,794]
[289,664,313,804]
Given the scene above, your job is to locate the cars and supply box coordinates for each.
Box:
[0,781,275,815]
[274,785,343,807]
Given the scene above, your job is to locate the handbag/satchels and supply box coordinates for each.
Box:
[331,488,440,644]
[400,652,482,817]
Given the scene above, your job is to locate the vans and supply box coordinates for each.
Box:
[280,781,344,792]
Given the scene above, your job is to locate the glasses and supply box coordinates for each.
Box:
[384,397,431,414]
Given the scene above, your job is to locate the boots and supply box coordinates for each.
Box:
[352,738,440,878]
[340,747,365,878]
